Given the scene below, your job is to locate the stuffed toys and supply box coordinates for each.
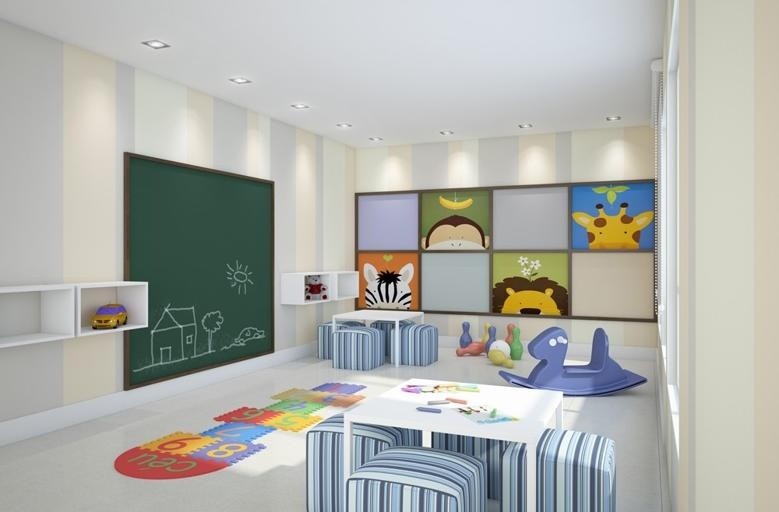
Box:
[305,275,327,300]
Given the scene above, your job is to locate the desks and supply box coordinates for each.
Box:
[332,309,424,368]
[344,376,564,512]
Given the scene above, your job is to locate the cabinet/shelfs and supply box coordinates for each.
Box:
[0,281,148,349]
[280,270,359,305]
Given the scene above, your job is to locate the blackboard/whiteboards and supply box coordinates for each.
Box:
[123,152,277,392]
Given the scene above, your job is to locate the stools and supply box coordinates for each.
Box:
[344,445,487,511]
[501,427,616,512]
[317,321,438,371]
[307,411,407,512]
